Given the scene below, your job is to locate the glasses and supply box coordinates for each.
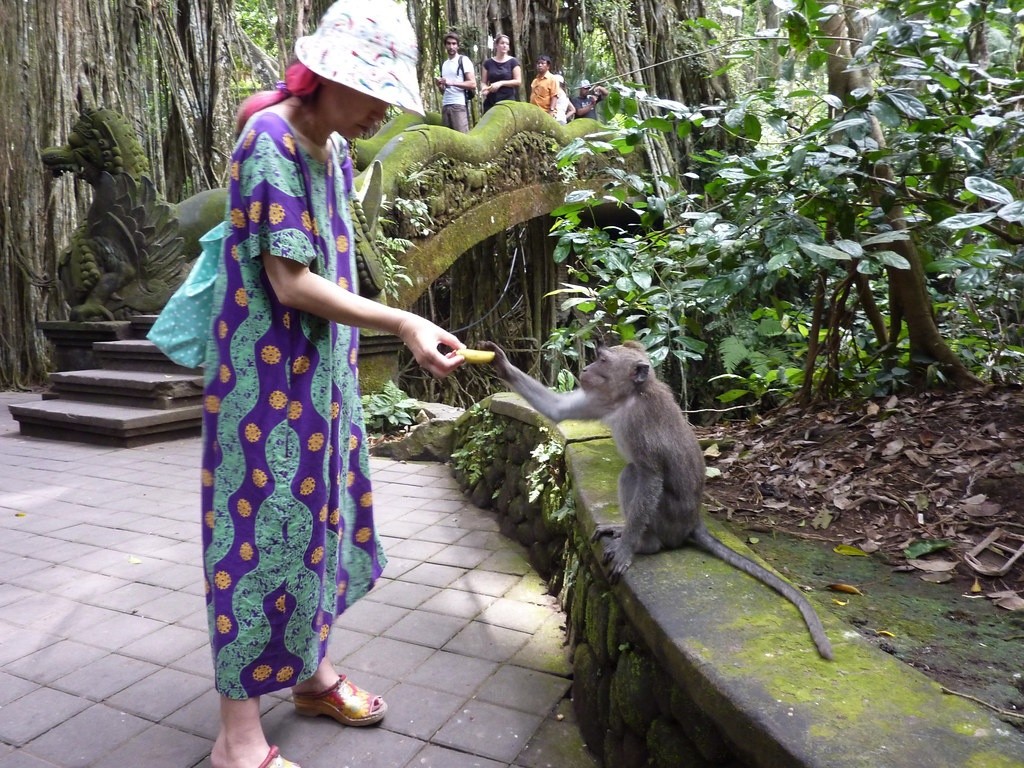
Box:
[445,41,457,45]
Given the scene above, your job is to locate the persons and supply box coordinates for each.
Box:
[434,32,476,134]
[573,79,609,121]
[198,0,467,768]
[530,55,558,121]
[481,35,522,115]
[551,74,576,126]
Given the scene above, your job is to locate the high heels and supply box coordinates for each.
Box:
[291,673,388,726]
[258,745,301,768]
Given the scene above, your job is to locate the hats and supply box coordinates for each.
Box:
[574,80,591,89]
[295,0,426,119]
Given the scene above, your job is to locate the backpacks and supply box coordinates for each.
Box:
[458,55,476,101]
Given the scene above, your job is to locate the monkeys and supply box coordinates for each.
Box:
[478,337,834,660]
